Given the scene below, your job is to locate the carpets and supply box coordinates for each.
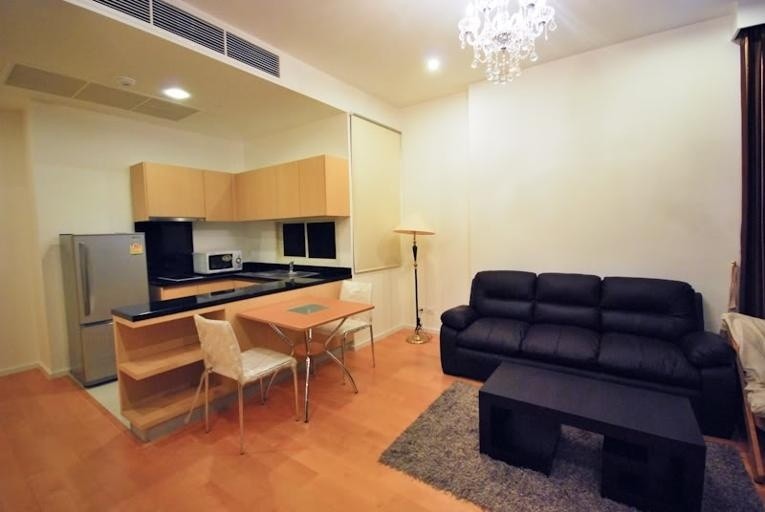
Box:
[377,380,765,512]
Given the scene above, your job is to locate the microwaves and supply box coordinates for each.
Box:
[196,288,244,303]
[193,250,243,274]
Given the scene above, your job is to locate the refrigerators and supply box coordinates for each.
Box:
[59,232,150,387]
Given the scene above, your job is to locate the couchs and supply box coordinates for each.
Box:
[440,271,741,440]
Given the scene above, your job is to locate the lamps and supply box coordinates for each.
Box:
[457,0,557,86]
[393,215,436,343]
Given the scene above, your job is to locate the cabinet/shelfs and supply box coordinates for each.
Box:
[114,305,226,431]
[277,154,350,219]
[198,169,235,223]
[130,161,206,222]
[235,166,276,221]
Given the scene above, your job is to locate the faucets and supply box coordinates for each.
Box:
[288,260,296,274]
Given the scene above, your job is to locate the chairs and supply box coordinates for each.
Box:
[193,313,299,454]
[307,281,376,385]
